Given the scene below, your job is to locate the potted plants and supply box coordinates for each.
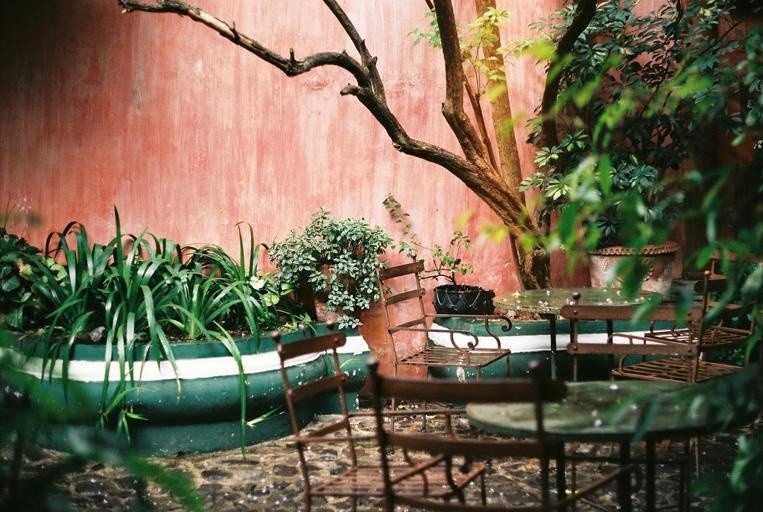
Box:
[516,1,763,302]
[385,191,495,314]
[267,207,394,329]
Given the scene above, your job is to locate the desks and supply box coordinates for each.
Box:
[494,286,662,381]
[466,381,758,511]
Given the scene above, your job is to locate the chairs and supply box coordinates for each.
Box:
[277,334,488,512]
[368,358,637,512]
[561,247,763,512]
[373,254,512,433]
